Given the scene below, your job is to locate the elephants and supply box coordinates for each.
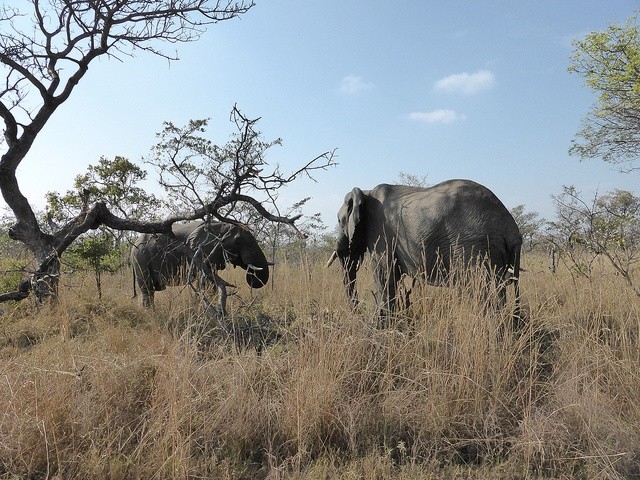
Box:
[321,178,524,334]
[129,219,277,309]
